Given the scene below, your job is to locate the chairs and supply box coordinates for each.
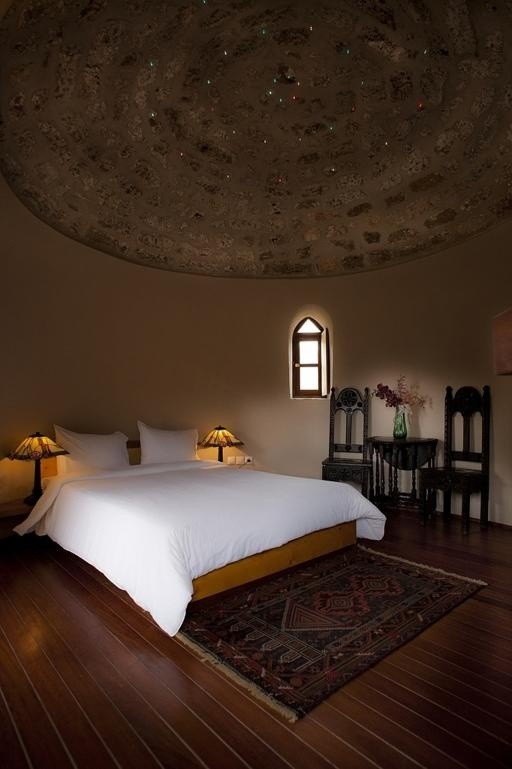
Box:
[322,387,372,498]
[419,385,491,537]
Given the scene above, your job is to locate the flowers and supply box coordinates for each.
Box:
[373,373,426,409]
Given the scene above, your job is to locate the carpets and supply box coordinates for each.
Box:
[174,543,489,723]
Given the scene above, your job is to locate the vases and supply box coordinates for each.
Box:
[391,404,408,438]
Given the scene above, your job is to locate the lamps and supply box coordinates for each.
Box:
[6,432,70,505]
[197,424,245,462]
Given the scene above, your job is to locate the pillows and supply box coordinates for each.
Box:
[137,420,200,464]
[53,424,129,476]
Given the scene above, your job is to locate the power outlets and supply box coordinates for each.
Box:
[245,456,254,464]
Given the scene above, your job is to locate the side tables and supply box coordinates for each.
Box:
[369,436,438,520]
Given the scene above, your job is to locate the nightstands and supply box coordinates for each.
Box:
[0,499,34,538]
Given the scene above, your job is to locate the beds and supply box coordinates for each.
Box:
[14,420,386,637]
